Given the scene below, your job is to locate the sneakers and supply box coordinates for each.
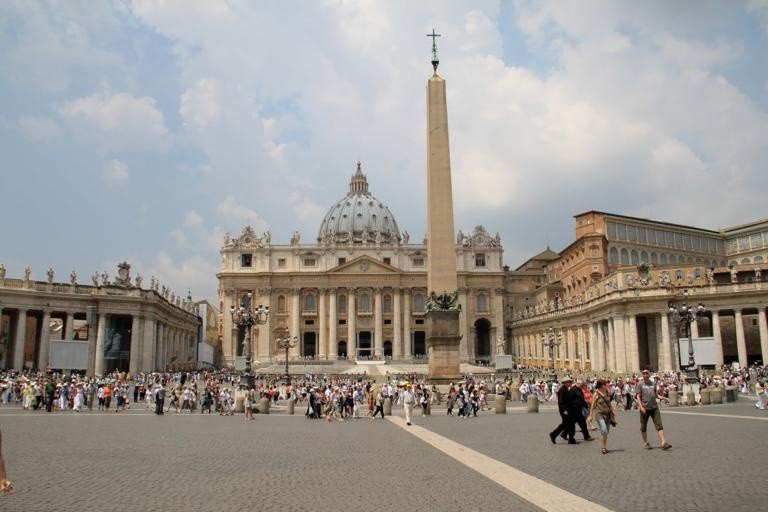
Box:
[663,443,672,450]
[549,426,597,444]
[643,442,652,450]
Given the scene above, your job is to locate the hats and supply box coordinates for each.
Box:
[641,370,651,377]
[561,377,573,383]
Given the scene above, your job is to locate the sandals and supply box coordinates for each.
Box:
[601,448,609,454]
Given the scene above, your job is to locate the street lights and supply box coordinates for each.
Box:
[275,335,299,377]
[540,326,563,368]
[229,288,270,373]
[666,289,708,366]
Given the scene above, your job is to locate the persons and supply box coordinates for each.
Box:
[0,366,555,425]
[299,355,420,361]
[0,434,14,494]
[634,370,672,450]
[486,358,490,366]
[549,376,617,453]
[555,362,768,410]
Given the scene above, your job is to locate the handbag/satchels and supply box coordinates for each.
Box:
[608,410,617,427]
